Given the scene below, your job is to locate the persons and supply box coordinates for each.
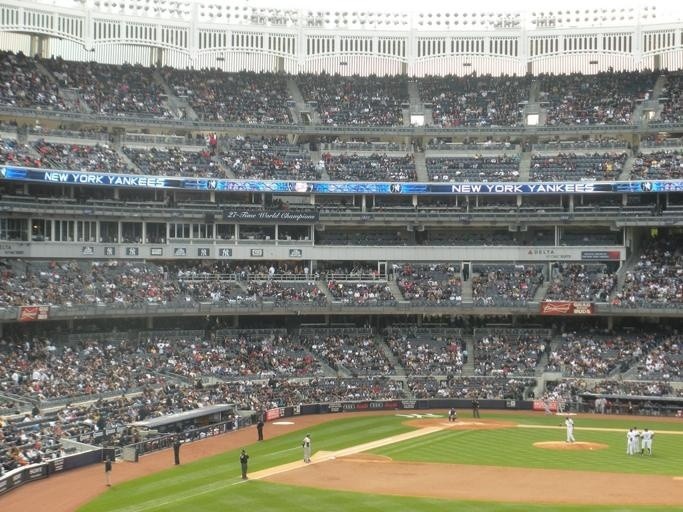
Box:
[0,49,683,482]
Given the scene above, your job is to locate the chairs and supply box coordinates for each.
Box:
[0,46,683,500]
